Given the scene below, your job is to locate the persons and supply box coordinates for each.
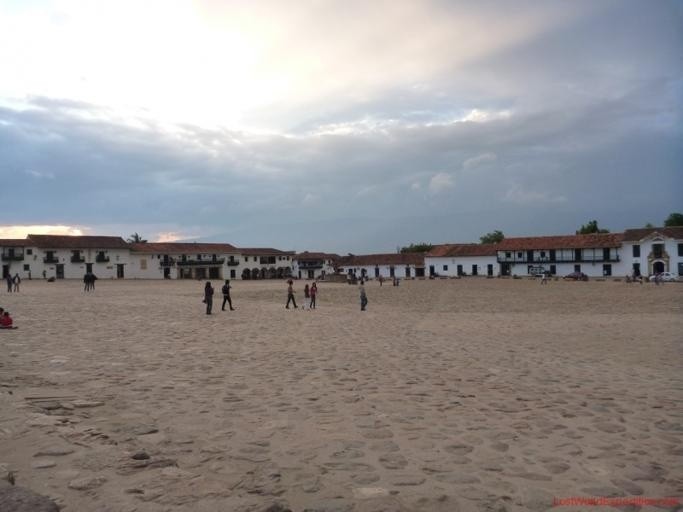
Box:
[203,280,214,315]
[629,269,665,286]
[303,283,310,309]
[308,280,317,309]
[0,306,18,329]
[88,271,97,290]
[220,280,234,311]
[357,279,369,312]
[284,279,299,310]
[5,274,12,292]
[541,270,547,285]
[0,311,12,329]
[603,266,607,279]
[82,270,89,292]
[378,273,383,286]
[12,272,20,293]
